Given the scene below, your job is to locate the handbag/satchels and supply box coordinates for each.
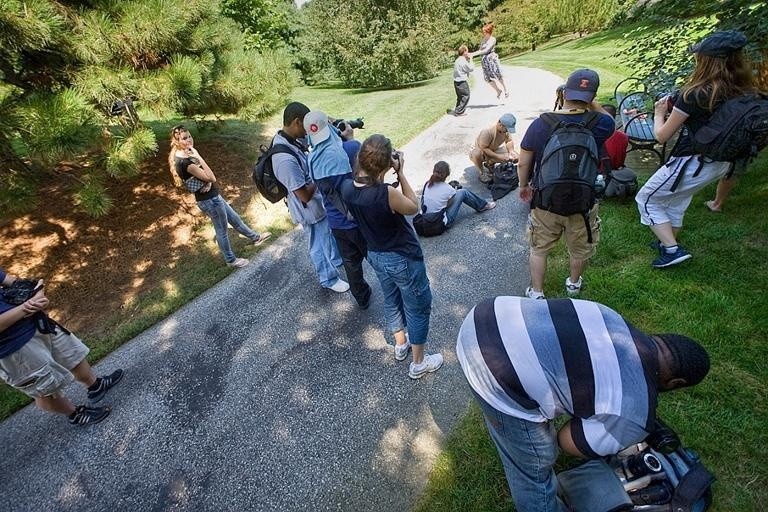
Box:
[604,165,640,204]
[412,210,447,240]
[178,156,210,195]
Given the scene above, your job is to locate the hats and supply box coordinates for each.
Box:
[302,110,333,149]
[689,28,753,60]
[563,67,602,107]
[498,112,517,135]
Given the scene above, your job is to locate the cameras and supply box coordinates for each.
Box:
[392,150,400,161]
[334,118,364,131]
[656,92,678,106]
[449,180,462,189]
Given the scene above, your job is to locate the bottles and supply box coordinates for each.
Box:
[594,173,606,201]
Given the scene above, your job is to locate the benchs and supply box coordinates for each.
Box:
[613,75,670,169]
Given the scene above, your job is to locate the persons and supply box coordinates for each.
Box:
[635,28,752,269]
[168,125,272,269]
[705,160,748,211]
[452,45,472,115]
[457,294,711,511]
[341,134,444,378]
[411,159,495,235]
[1,268,122,425]
[588,102,628,185]
[466,23,510,101]
[471,113,518,183]
[272,100,408,328]
[517,69,611,300]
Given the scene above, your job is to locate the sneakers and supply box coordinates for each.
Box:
[478,170,491,184]
[393,331,413,362]
[86,367,126,404]
[483,162,496,175]
[407,352,445,380]
[646,238,666,253]
[564,275,584,298]
[67,403,113,428]
[319,278,352,295]
[650,245,693,270]
[479,201,497,213]
[524,285,546,301]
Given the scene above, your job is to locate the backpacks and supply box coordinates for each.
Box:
[251,133,305,205]
[530,111,603,218]
[553,443,720,512]
[686,80,768,163]
[487,163,519,202]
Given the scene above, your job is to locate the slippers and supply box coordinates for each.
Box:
[496,89,511,98]
[253,231,273,247]
[224,257,250,269]
[704,200,723,213]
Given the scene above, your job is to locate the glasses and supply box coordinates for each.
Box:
[391,148,402,176]
